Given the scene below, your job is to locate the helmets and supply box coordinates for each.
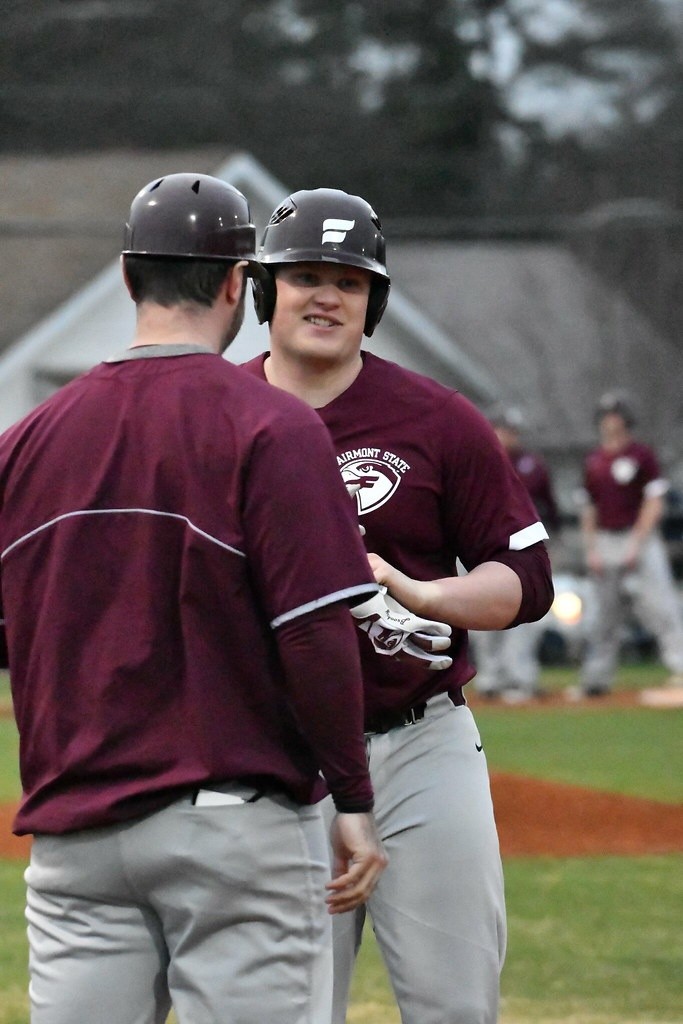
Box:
[122,173,259,262]
[251,188,391,338]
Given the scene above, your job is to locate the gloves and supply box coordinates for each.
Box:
[350,585,452,671]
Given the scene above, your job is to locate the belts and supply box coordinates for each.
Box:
[366,685,465,735]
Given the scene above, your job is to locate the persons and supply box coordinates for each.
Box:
[477,390,683,697]
[1,170,553,1024]
